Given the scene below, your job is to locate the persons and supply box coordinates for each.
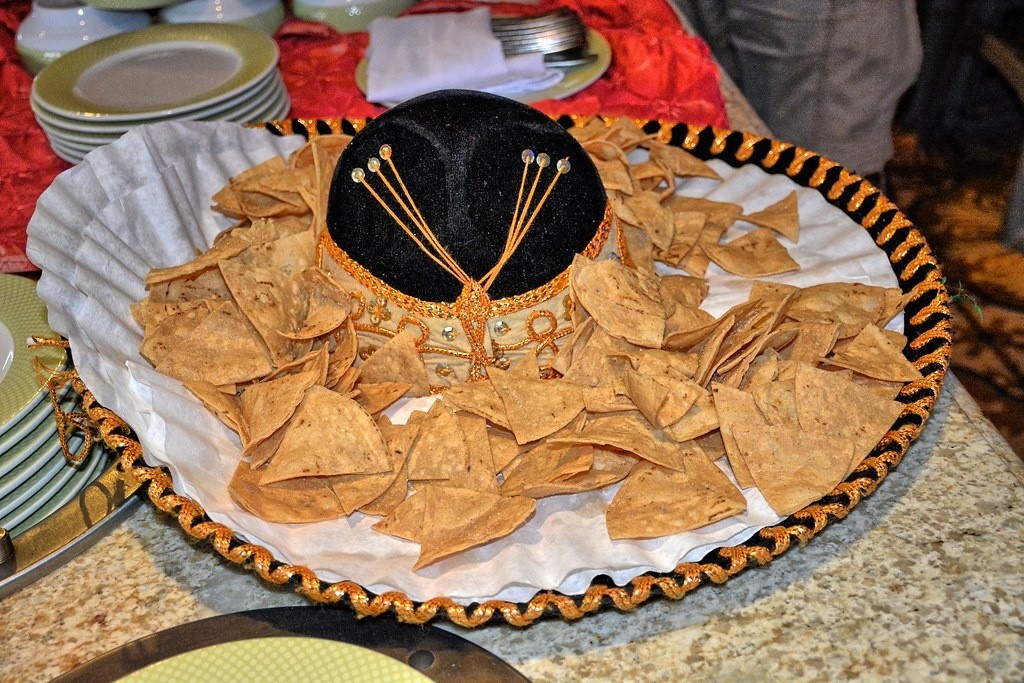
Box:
[671,0,922,194]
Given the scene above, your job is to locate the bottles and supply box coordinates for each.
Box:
[15,0,415,75]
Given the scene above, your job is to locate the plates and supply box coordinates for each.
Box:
[0,271,107,535]
[354,28,611,104]
[29,22,291,165]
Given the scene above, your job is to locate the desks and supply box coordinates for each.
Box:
[0,0,1024,683]
